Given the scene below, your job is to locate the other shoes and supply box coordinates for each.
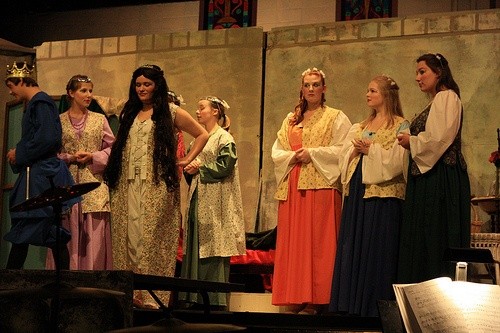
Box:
[297,306,322,315]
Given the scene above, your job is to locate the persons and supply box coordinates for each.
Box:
[270,66,354,315]
[44,73,117,270]
[396,52,471,284]
[102,64,211,309]
[4,60,84,271]
[329,74,412,317]
[166,91,188,316]
[177,95,248,313]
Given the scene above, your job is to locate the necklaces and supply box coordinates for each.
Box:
[141,119,146,123]
[68,109,88,129]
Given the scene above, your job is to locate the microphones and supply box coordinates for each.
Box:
[369,132,372,135]
[300,127,303,128]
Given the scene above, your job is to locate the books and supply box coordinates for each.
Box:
[391,277,500,333]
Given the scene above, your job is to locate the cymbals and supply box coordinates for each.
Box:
[12,182,100,211]
